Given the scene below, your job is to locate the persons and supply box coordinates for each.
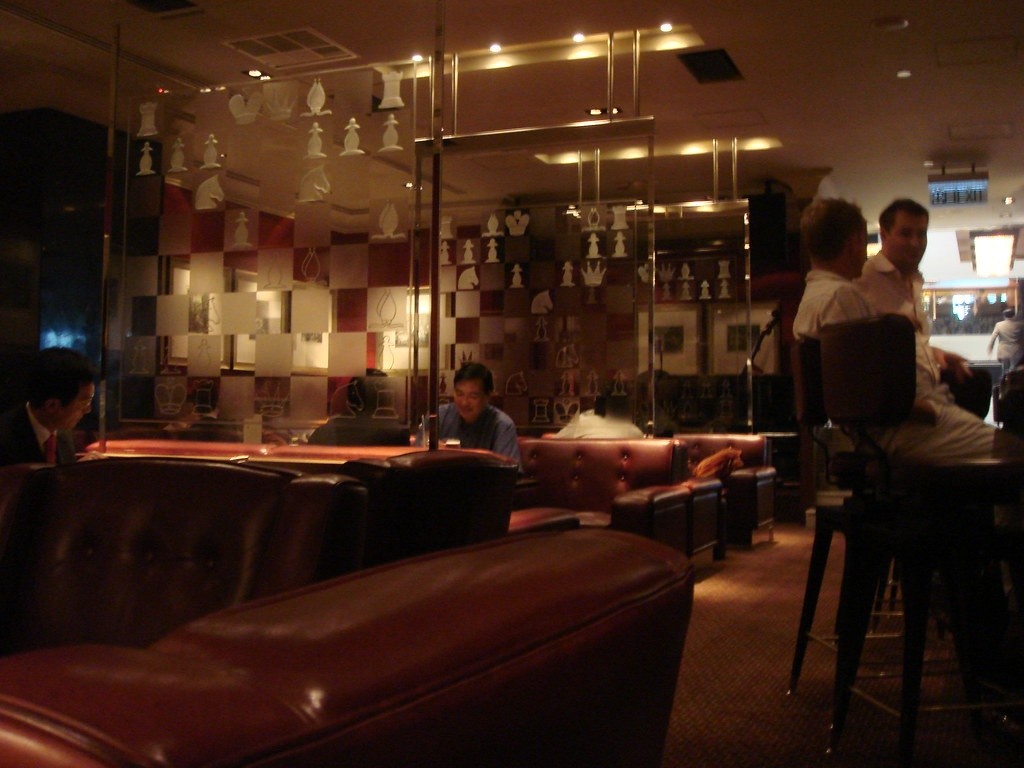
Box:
[986,308,1024,382]
[0,346,101,471]
[160,366,411,446]
[791,199,1024,616]
[414,363,526,483]
[851,196,976,408]
[553,377,650,441]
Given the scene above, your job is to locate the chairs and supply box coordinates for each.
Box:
[820,313,1024,768]
[787,337,964,698]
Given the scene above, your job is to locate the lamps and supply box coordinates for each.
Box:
[928,165,989,206]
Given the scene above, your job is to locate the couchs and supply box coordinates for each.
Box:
[0,440,694,768]
[673,433,775,544]
[504,436,727,560]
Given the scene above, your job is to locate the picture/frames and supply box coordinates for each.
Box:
[705,303,779,374]
[635,303,704,377]
